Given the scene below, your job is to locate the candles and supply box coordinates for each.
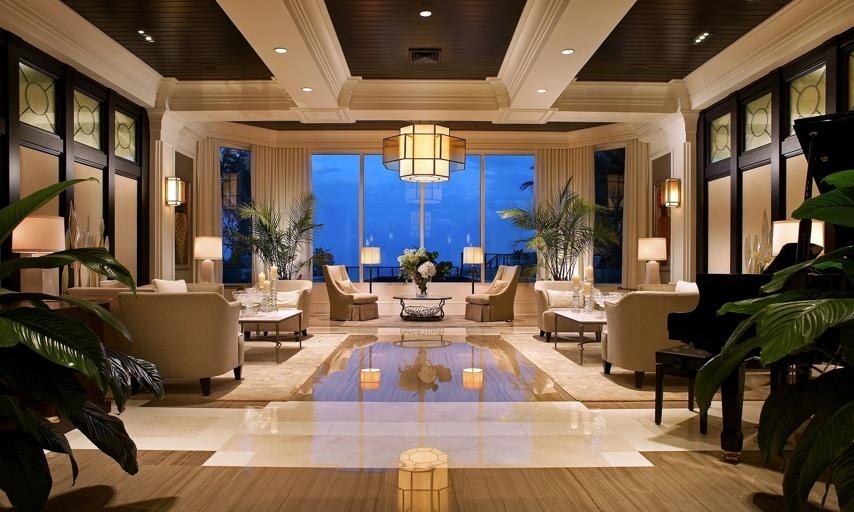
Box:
[583,282,593,314]
[571,274,582,313]
[269,261,280,312]
[258,271,266,312]
[585,265,594,285]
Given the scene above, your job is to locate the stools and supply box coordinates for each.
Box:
[655,343,720,435]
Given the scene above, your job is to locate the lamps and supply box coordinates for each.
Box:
[463,347,484,389]
[360,344,382,390]
[637,237,667,285]
[463,246,485,294]
[164,176,185,207]
[360,246,381,294]
[772,220,824,256]
[193,236,223,283]
[383,448,458,512]
[12,215,65,302]
[383,120,466,183]
[661,178,681,208]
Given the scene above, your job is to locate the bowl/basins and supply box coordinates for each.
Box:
[592,296,620,308]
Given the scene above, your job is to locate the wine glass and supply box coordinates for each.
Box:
[232,291,264,316]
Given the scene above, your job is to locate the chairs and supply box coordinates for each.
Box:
[464,264,521,322]
[601,291,699,388]
[534,280,602,342]
[320,335,379,377]
[119,291,244,396]
[323,265,378,321]
[244,280,313,342]
[465,335,521,378]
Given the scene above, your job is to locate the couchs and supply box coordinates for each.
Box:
[68,278,224,327]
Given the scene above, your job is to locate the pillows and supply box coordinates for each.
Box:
[674,280,700,293]
[99,280,137,288]
[492,280,509,294]
[336,280,352,295]
[152,278,188,293]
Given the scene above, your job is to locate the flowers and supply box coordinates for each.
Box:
[396,246,439,286]
[398,364,453,393]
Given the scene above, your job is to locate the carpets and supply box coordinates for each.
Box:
[127,333,353,401]
[499,332,724,401]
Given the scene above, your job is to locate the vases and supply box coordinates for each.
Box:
[414,348,431,366]
[410,276,431,297]
